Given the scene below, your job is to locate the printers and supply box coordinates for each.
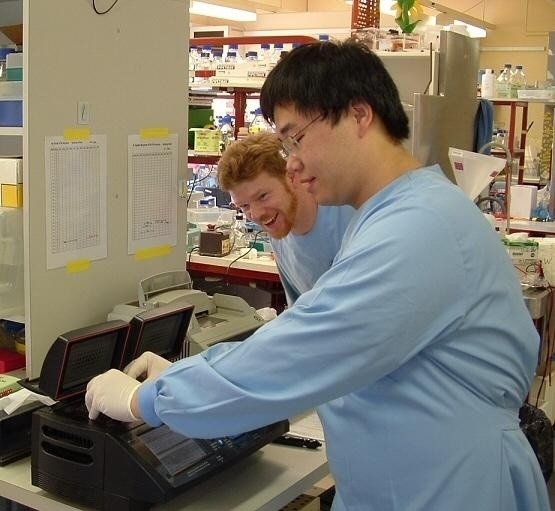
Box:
[109,269,266,358]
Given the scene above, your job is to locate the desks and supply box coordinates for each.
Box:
[522,288,554,397]
[0,408,338,511]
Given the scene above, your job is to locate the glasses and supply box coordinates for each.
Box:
[282,113,323,156]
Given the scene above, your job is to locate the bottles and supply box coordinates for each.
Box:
[230,215,249,247]
[188,33,330,88]
[480,63,527,99]
[218,114,234,153]
[248,108,270,136]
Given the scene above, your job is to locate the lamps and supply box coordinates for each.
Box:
[188,0,259,25]
[345,1,496,39]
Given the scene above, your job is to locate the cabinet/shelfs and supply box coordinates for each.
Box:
[188,30,481,319]
[482,101,530,202]
[0,1,190,385]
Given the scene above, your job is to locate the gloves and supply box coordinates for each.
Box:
[85,368,142,423]
[123,351,173,381]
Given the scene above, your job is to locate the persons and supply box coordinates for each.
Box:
[218,130,357,307]
[83,41,548,511]
